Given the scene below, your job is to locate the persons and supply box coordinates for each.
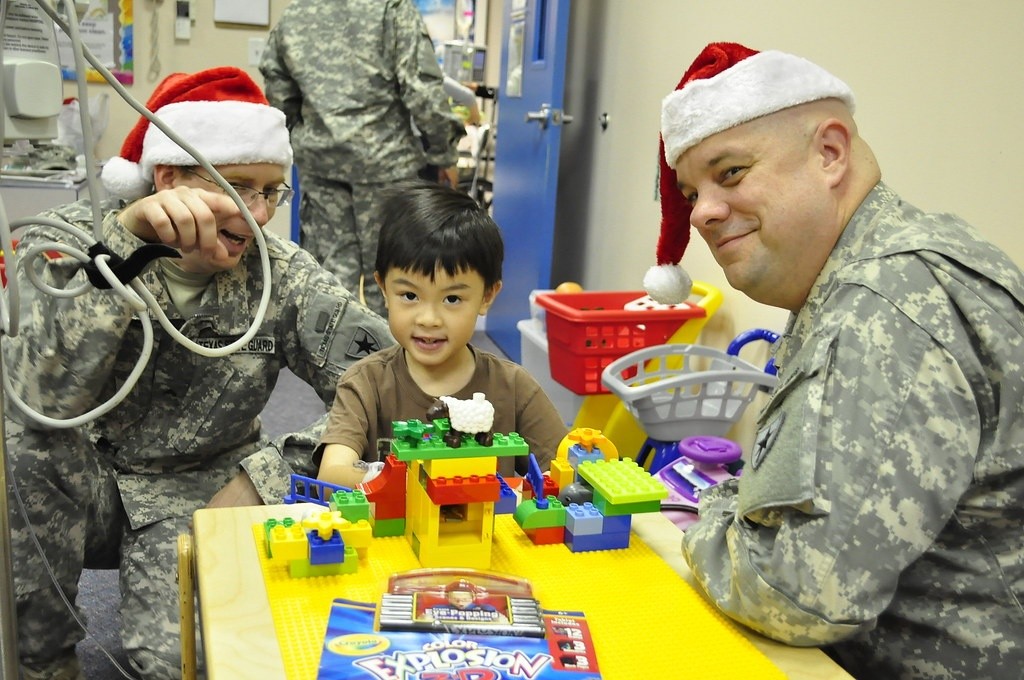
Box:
[641,41,1023,680]
[417,579,509,624]
[258,0,468,320]
[0,66,401,680]
[317,187,569,490]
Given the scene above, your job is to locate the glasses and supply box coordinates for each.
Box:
[178,163,295,210]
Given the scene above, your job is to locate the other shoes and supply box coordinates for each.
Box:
[21,644,83,680]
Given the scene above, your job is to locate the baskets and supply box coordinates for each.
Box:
[535,290,708,396]
[601,346,781,442]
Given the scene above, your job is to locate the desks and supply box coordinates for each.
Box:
[176,488,856,680]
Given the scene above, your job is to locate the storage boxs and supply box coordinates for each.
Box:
[516,319,585,426]
[535,292,708,397]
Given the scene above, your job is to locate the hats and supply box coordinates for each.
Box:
[99,65,295,200]
[643,41,857,304]
